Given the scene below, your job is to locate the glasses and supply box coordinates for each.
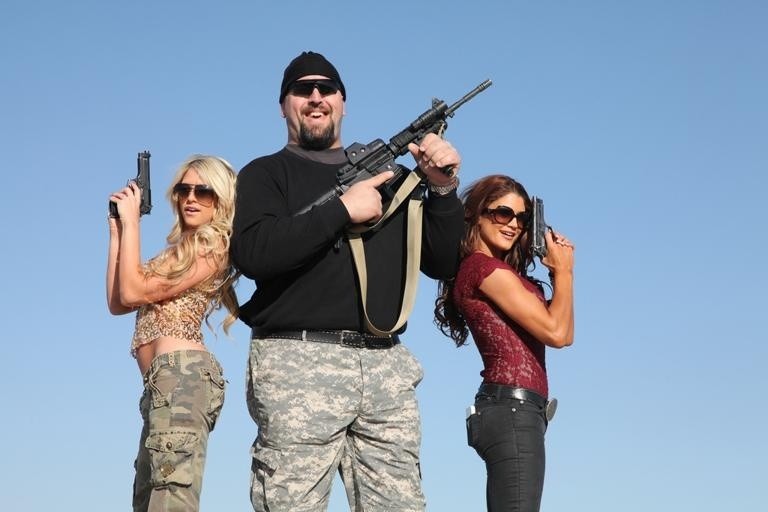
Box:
[173,183,215,202]
[288,79,338,96]
[482,206,530,230]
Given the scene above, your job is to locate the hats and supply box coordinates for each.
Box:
[279,51,346,101]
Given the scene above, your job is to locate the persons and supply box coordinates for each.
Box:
[227,50,465,512]
[104,153,242,511]
[432,174,576,512]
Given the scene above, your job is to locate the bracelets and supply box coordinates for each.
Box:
[429,177,460,196]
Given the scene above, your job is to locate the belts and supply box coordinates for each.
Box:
[476,384,557,420]
[252,327,399,348]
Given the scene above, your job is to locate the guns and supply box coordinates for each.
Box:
[530,197,554,259]
[294,81,493,249]
[109,150,151,218]
